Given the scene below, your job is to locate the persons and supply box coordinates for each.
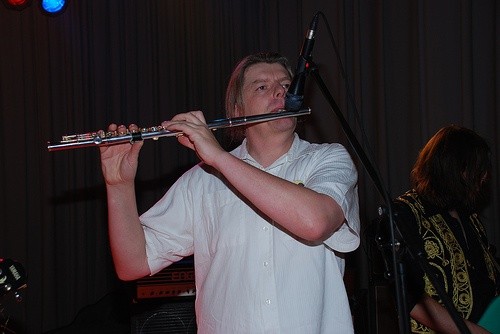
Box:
[375,125,500,334]
[98,51,360,334]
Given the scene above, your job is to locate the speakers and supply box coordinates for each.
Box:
[127,280,198,334]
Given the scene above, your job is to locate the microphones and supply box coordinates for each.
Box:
[284,11,320,113]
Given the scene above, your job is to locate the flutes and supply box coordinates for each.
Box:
[46,104,313,152]
